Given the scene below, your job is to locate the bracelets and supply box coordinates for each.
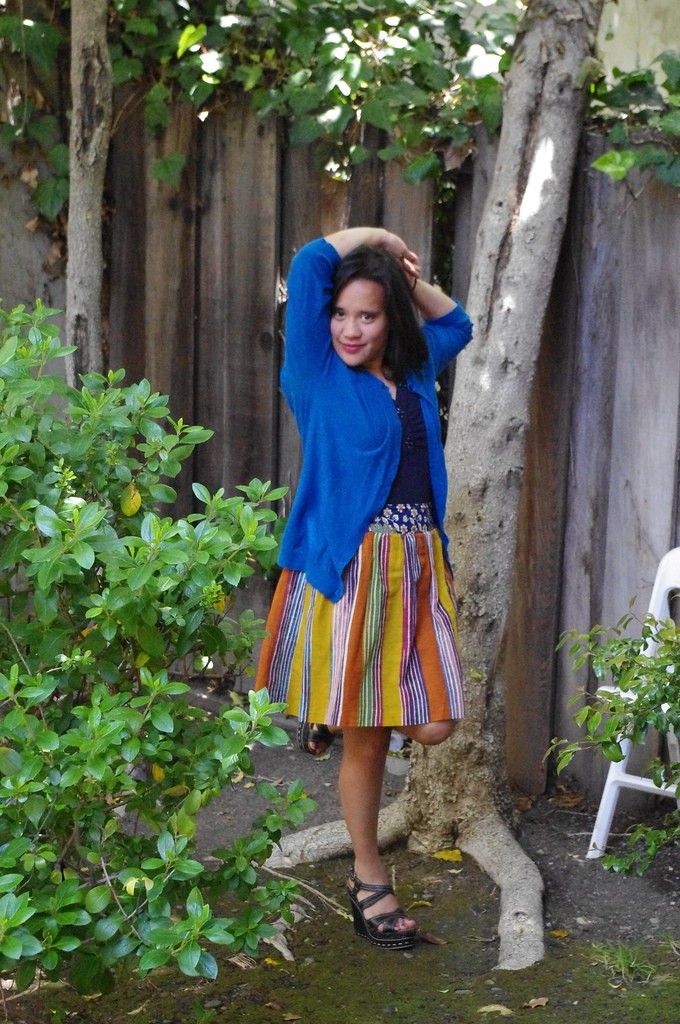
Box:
[411,277,417,292]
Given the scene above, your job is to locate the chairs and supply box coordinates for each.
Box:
[587,540,680,859]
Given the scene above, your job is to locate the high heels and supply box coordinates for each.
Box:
[298,720,336,756]
[346,866,416,949]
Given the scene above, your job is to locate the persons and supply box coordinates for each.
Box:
[253,228,472,950]
